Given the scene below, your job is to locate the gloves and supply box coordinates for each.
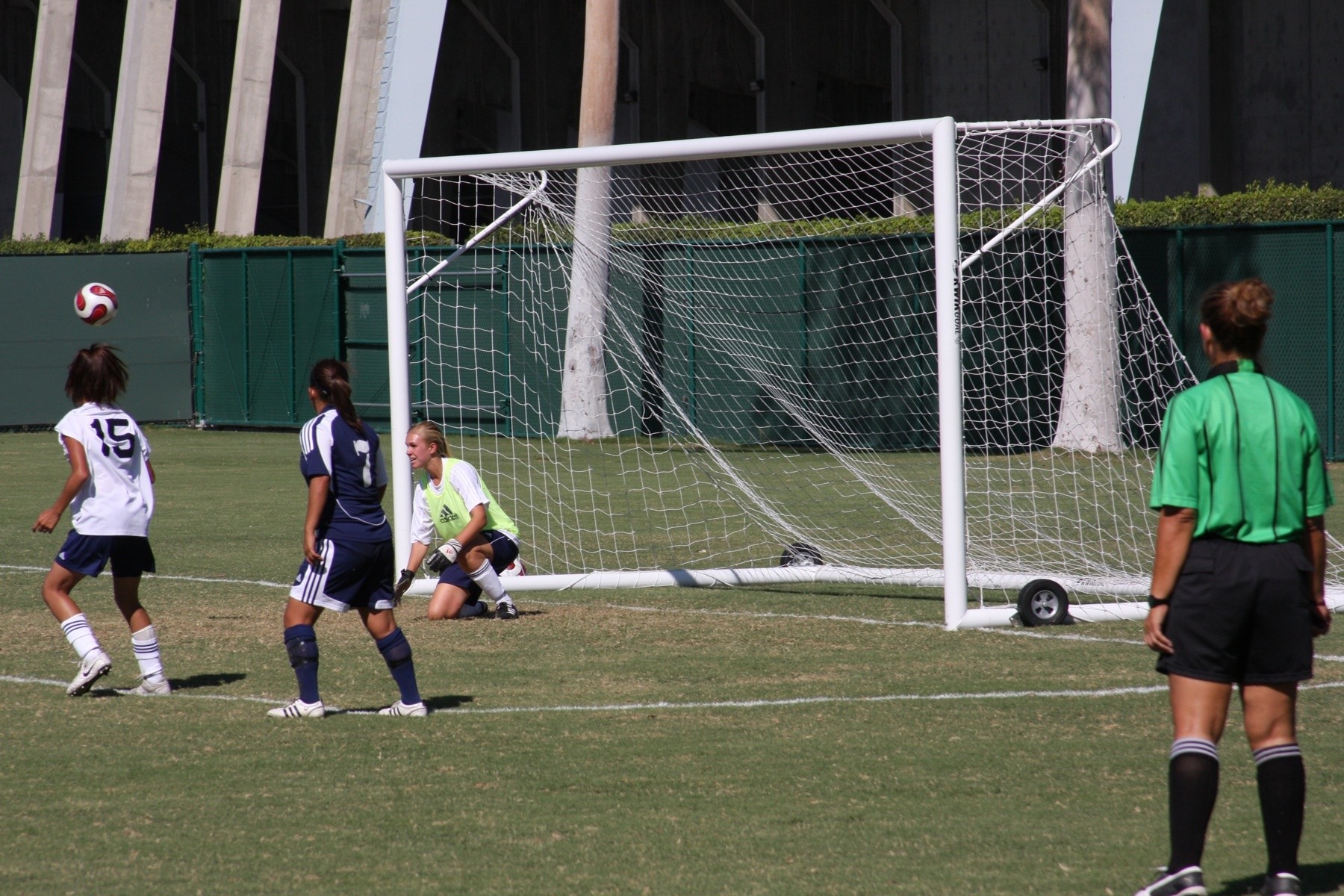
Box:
[427,538,462,577]
[394,568,414,607]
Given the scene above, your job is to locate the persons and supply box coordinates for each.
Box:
[1135,276,1331,896]
[262,357,428,719]
[28,345,172,696]
[393,420,520,620]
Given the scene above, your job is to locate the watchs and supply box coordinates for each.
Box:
[1148,594,1168,608]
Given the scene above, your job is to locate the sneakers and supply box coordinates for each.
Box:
[1134,865,1207,896]
[267,698,324,718]
[474,600,488,617]
[66,653,112,696]
[1266,871,1302,896]
[379,700,427,717]
[493,599,518,619]
[111,674,171,696]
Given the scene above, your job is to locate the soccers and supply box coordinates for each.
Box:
[74,283,117,327]
